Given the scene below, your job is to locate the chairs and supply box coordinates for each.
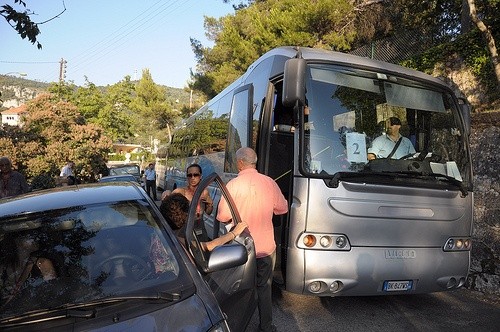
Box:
[78,205,156,276]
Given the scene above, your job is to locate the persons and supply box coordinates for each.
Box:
[128,193,248,284]
[103,154,111,164]
[60,160,75,177]
[140,163,161,202]
[217,147,288,332]
[368,117,417,163]
[0,230,57,297]
[161,164,214,260]
[0,157,29,198]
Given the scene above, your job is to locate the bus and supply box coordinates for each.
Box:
[155,142,164,191]
[165,45,475,297]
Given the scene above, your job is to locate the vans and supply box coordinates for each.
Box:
[107,163,145,188]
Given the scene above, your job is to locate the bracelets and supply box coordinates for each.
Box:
[208,201,214,208]
[229,231,237,240]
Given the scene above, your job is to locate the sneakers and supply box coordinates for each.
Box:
[260,324,277,332]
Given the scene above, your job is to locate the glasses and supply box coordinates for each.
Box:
[187,173,200,178]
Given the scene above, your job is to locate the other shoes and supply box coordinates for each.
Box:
[154,198,160,201]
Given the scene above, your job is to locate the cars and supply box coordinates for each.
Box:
[0,173,257,332]
[97,174,137,183]
